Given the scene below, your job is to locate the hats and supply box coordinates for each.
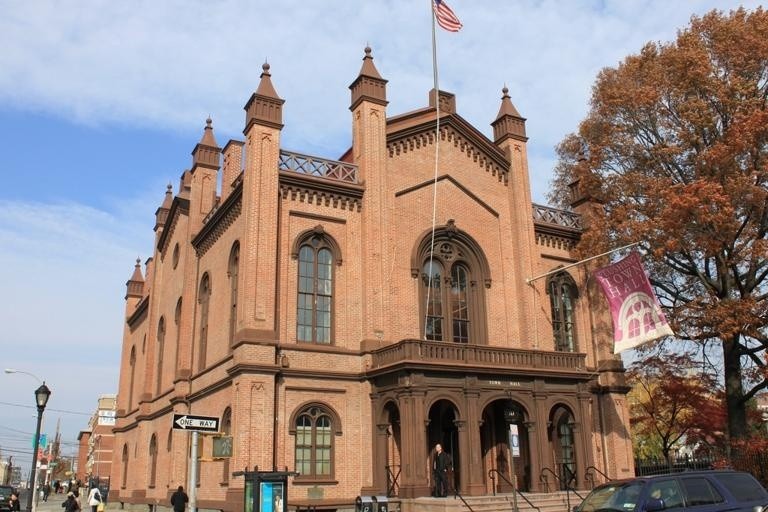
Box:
[67,491,76,498]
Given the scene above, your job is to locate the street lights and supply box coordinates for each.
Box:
[24,380,52,512]
[5,368,44,387]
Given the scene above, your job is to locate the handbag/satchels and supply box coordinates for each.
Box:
[94,493,100,502]
[97,503,105,512]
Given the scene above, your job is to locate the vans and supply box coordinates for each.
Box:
[569,465,768,512]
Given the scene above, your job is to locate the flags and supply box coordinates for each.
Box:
[591,251,675,356]
[432,0,463,33]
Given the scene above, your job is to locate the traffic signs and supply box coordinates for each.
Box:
[172,412,220,434]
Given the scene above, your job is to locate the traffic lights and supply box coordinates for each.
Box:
[212,436,233,458]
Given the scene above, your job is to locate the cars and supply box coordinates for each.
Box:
[25,478,74,489]
[11,481,22,489]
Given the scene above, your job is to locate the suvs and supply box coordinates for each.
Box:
[0,486,19,511]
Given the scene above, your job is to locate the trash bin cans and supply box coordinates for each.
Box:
[355,495,388,512]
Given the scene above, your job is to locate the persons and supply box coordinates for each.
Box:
[87,485,102,512]
[274,495,282,512]
[170,486,188,512]
[645,485,666,510]
[7,493,20,512]
[432,444,449,498]
[43,476,83,512]
